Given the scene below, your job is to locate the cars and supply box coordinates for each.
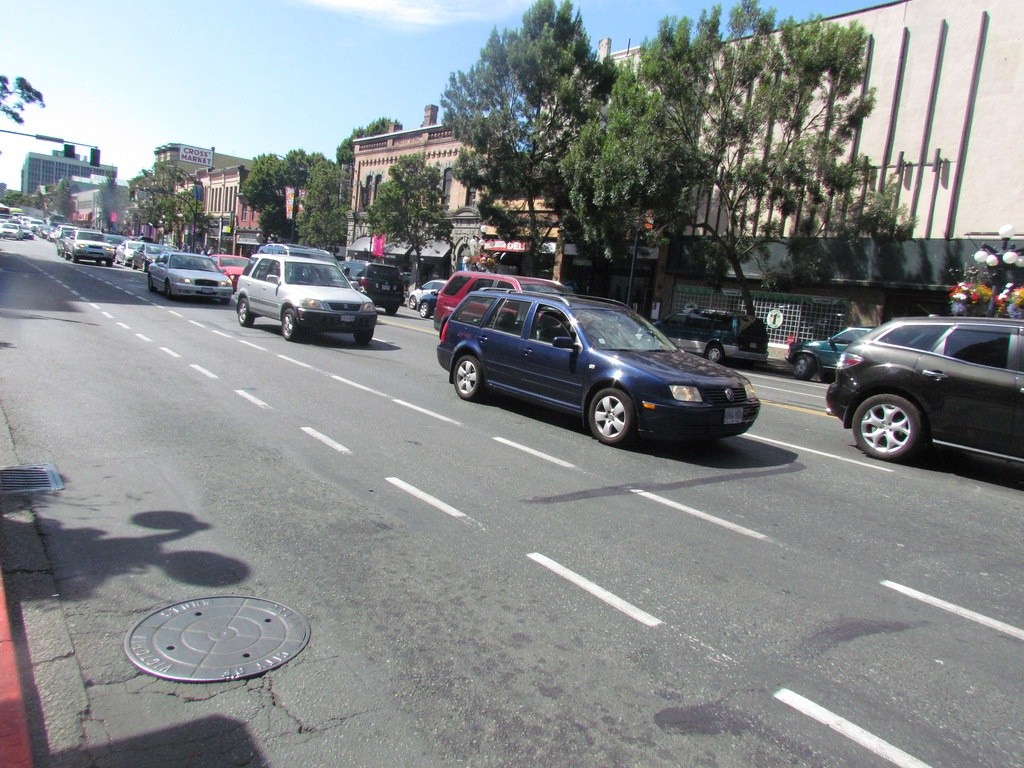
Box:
[408,280,448,310]
[57,230,73,260]
[0,223,24,240]
[106,234,129,260]
[20,224,34,240]
[785,325,874,383]
[37,214,89,244]
[132,243,176,272]
[437,288,761,446]
[209,254,250,293]
[147,250,234,306]
[0,203,44,232]
[65,230,115,267]
[130,236,154,244]
[258,243,341,270]
[234,253,378,345]
[115,240,147,266]
[434,270,579,330]
[826,313,1024,465]
[416,288,441,318]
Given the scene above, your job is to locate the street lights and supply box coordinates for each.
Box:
[973,225,1024,317]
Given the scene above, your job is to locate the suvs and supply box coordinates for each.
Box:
[650,306,768,369]
[339,261,406,314]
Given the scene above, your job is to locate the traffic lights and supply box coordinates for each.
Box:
[130,188,136,203]
